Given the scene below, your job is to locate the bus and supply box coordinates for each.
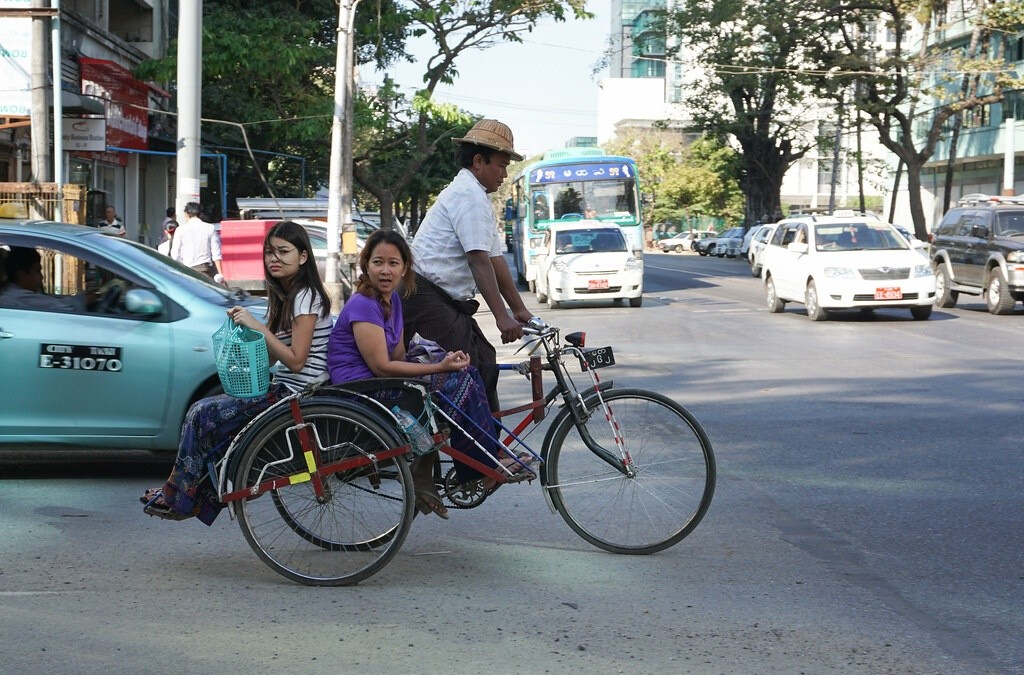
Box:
[503,197,515,252]
[509,147,645,292]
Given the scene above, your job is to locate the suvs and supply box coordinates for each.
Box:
[537,220,643,308]
[658,229,718,254]
[762,208,938,322]
[929,193,1024,315]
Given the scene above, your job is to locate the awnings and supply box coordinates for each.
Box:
[81,57,173,99]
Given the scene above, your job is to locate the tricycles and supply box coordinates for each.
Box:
[203,316,719,585]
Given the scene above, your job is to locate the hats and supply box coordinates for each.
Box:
[450,119,523,163]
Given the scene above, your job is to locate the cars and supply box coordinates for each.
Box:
[0,216,299,483]
[892,226,931,261]
[695,224,776,276]
[235,195,418,283]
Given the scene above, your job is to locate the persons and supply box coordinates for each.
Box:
[387,117,547,521]
[789,225,839,252]
[155,220,181,255]
[172,202,225,285]
[137,219,335,527]
[97,204,128,239]
[0,245,125,317]
[160,206,177,236]
[328,227,539,494]
[532,190,624,222]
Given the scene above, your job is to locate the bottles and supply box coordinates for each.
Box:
[391,405,435,452]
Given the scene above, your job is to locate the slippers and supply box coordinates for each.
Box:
[396,476,449,519]
[487,459,531,496]
[140,489,171,512]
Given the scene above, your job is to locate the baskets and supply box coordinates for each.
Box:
[212,316,270,398]
